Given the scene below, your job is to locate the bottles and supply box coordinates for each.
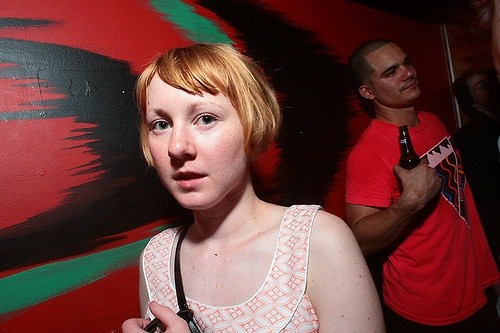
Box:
[399,125,420,170]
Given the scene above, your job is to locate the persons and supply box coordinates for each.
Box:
[121,41,386,333]
[342,0,500,333]
